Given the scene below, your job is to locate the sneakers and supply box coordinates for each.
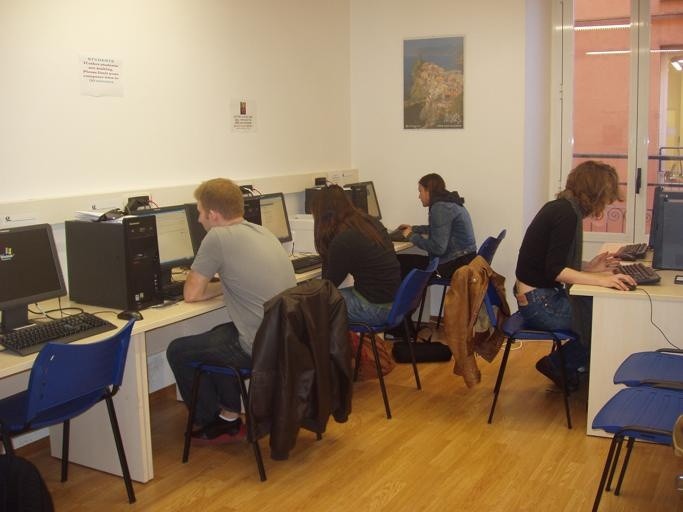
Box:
[184,416,245,445]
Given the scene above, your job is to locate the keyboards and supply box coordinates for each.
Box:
[0,312,117,356]
[388,224,409,242]
[164,277,220,301]
[290,254,323,274]
[614,243,647,259]
[613,263,661,285]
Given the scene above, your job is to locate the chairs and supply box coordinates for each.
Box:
[3,230,510,510]
[604,344,683,493]
[582,386,683,510]
[466,255,582,430]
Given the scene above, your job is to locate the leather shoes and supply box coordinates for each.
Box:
[536,355,579,391]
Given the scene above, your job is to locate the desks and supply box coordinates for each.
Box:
[568,239,682,447]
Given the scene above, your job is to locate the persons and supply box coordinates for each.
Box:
[166,179,298,448]
[385,174,477,341]
[314,184,402,330]
[513,161,638,393]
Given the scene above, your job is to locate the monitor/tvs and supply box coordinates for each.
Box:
[0,223,67,334]
[253,191,292,244]
[343,180,382,220]
[135,205,208,287]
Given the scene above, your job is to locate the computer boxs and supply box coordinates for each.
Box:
[305,184,368,229]
[649,186,683,270]
[65,214,164,310]
[185,198,262,254]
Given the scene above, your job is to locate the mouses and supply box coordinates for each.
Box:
[616,253,636,261]
[118,310,143,321]
[613,281,636,291]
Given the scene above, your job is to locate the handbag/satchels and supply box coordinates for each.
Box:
[357,334,397,382]
[390,325,451,364]
